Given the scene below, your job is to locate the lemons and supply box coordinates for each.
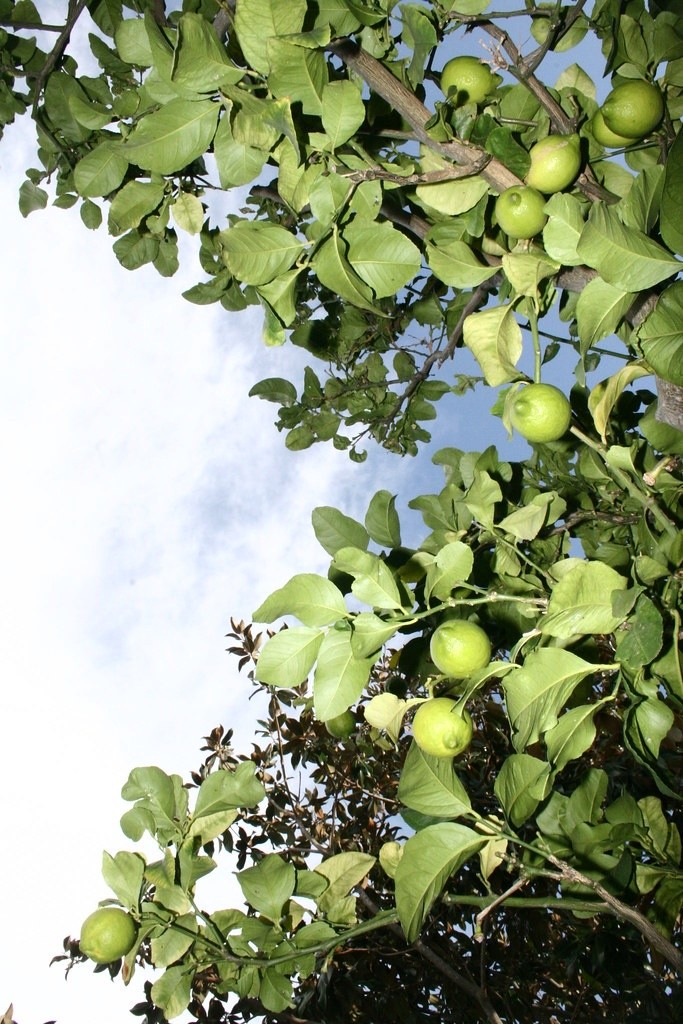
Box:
[76,9,668,964]
